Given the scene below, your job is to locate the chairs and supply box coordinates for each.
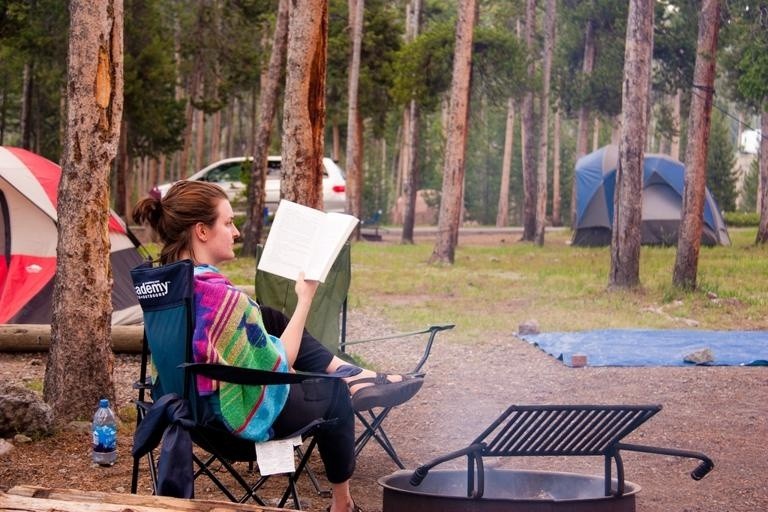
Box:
[247,245,456,498]
[130,255,362,511]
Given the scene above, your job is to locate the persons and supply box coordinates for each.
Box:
[131,180,425,512]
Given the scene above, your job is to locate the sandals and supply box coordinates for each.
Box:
[349,371,424,411]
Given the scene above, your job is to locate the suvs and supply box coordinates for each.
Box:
[152,156,351,230]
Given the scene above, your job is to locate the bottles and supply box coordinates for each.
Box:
[92,398,118,465]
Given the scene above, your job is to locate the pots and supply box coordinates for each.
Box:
[377,469,640,512]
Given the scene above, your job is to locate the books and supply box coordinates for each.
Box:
[257,198,359,283]
